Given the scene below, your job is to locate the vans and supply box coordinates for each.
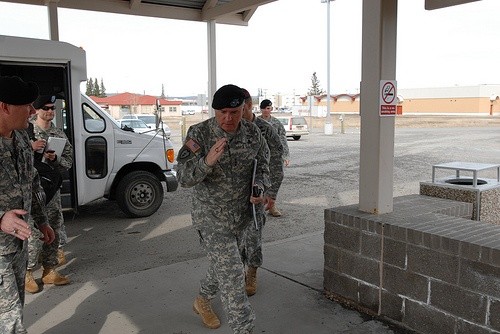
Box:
[0,35,179,221]
[182,109,196,115]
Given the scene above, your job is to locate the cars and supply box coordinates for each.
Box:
[117,120,165,138]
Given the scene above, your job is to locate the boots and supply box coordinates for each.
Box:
[245,265,257,296]
[40,269,68,285]
[267,199,282,216]
[56,249,65,266]
[23,270,39,293]
[194,295,221,329]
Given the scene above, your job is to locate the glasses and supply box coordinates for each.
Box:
[40,105,56,111]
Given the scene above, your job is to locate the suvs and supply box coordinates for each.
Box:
[120,114,171,140]
[277,117,309,139]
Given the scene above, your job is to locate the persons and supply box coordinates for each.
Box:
[176,85,272,334]
[0,76,73,334]
[241,88,289,295]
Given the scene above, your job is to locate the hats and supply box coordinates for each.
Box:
[213,84,245,110]
[0,76,40,105]
[32,95,57,109]
[260,100,273,109]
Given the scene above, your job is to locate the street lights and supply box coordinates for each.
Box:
[321,0,336,135]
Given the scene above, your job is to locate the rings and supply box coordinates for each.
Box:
[215,148,218,153]
[13,228,17,234]
